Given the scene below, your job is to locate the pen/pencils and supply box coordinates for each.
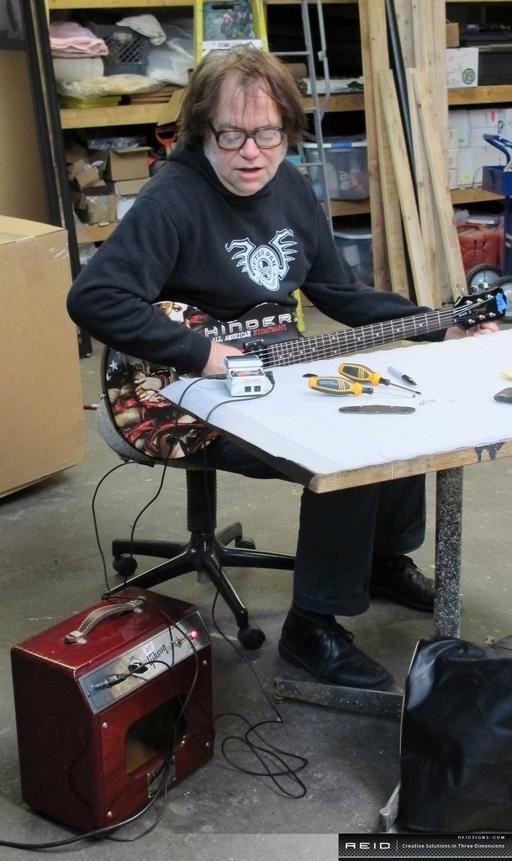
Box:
[387,365,418,387]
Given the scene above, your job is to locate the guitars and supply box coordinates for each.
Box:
[98,288,507,462]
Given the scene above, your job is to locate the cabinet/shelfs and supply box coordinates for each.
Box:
[19,1,511,358]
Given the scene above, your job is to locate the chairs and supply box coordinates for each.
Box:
[95,336,295,651]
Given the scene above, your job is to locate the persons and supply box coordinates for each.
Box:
[67,44,502,688]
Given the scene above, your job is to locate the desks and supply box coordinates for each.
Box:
[163,329,512,833]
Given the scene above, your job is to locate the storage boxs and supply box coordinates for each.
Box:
[303,138,370,203]
[0,214,85,499]
[334,228,375,283]
[65,133,153,225]
[446,22,460,48]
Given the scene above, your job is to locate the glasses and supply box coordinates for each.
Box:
[207,126,288,151]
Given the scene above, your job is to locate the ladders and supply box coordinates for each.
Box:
[269,1,334,241]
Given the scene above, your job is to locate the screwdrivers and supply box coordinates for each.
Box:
[336,358,422,397]
[307,376,415,397]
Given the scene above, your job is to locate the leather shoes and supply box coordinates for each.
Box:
[368,555,435,613]
[279,603,393,690]
